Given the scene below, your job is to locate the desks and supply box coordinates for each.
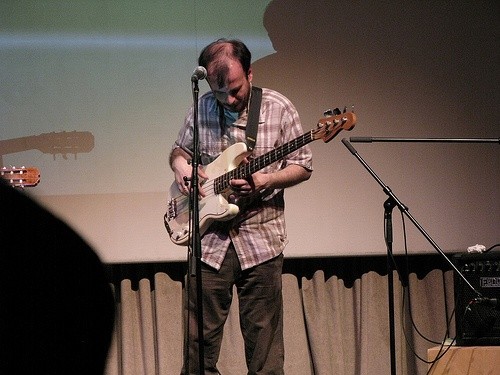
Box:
[427,344,500,375]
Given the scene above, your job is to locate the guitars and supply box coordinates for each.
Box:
[1,166,42,189]
[164,103,357,245]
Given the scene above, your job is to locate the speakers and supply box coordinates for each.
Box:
[454,250,500,347]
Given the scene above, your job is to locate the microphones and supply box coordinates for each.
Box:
[472,298,497,305]
[190,65,207,82]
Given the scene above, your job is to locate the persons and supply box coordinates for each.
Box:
[168,39,314,375]
